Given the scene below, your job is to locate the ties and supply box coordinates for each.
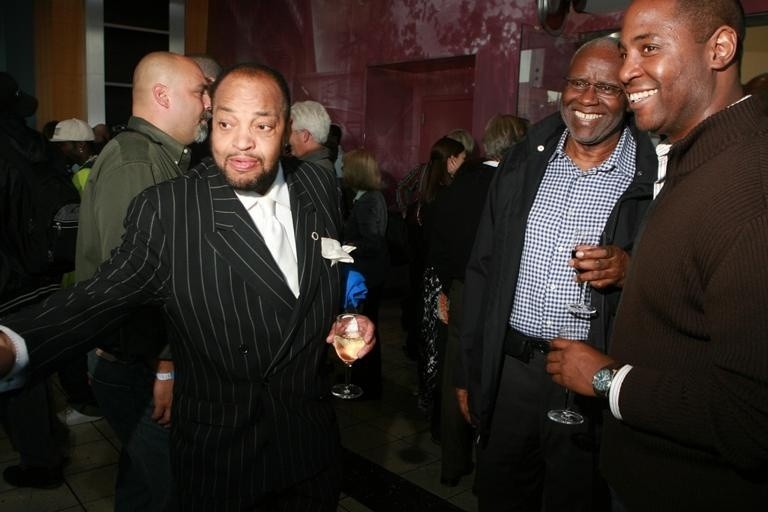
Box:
[257,197,300,299]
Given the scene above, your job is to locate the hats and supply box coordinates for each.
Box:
[0,72,39,117]
[49,117,95,142]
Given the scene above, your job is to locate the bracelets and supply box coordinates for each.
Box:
[151,369,177,383]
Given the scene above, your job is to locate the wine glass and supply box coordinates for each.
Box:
[545,325,593,424]
[566,228,603,318]
[333,315,367,399]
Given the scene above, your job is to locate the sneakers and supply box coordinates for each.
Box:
[3,406,104,489]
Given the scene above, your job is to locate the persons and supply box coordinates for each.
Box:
[736,69,767,110]
[441,37,658,510]
[0,62,379,511]
[1,52,532,512]
[546,0,768,511]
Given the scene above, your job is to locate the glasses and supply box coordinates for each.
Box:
[566,78,622,96]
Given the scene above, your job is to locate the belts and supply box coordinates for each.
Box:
[512,330,585,353]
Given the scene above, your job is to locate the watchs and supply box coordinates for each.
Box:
[588,361,626,410]
